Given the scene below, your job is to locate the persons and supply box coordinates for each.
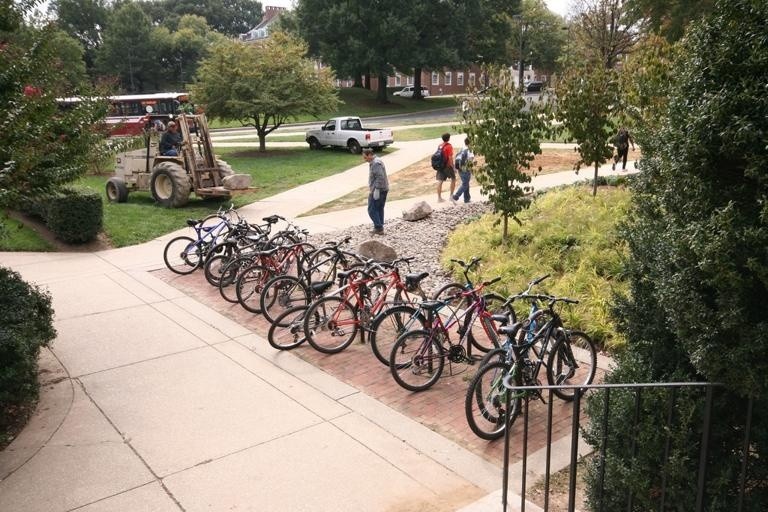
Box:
[611,127,636,172]
[462,97,470,123]
[431,133,478,203]
[361,147,389,235]
[159,120,183,156]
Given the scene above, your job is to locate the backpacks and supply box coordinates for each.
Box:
[431,143,450,170]
[455,148,468,169]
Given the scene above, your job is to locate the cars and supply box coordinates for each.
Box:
[517,81,544,92]
[477,86,491,95]
[393,86,429,98]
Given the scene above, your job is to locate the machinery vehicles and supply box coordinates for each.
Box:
[106,112,259,207]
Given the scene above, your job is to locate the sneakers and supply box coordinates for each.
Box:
[449,191,459,200]
[369,229,384,235]
[438,199,445,203]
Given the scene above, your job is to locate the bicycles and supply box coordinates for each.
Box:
[163,202,597,440]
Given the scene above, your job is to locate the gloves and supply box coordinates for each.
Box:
[373,188,380,200]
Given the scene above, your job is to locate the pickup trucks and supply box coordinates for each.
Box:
[305,116,393,154]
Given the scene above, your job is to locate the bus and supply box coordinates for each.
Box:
[48,93,200,137]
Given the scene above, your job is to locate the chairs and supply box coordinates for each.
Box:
[353,123,360,128]
